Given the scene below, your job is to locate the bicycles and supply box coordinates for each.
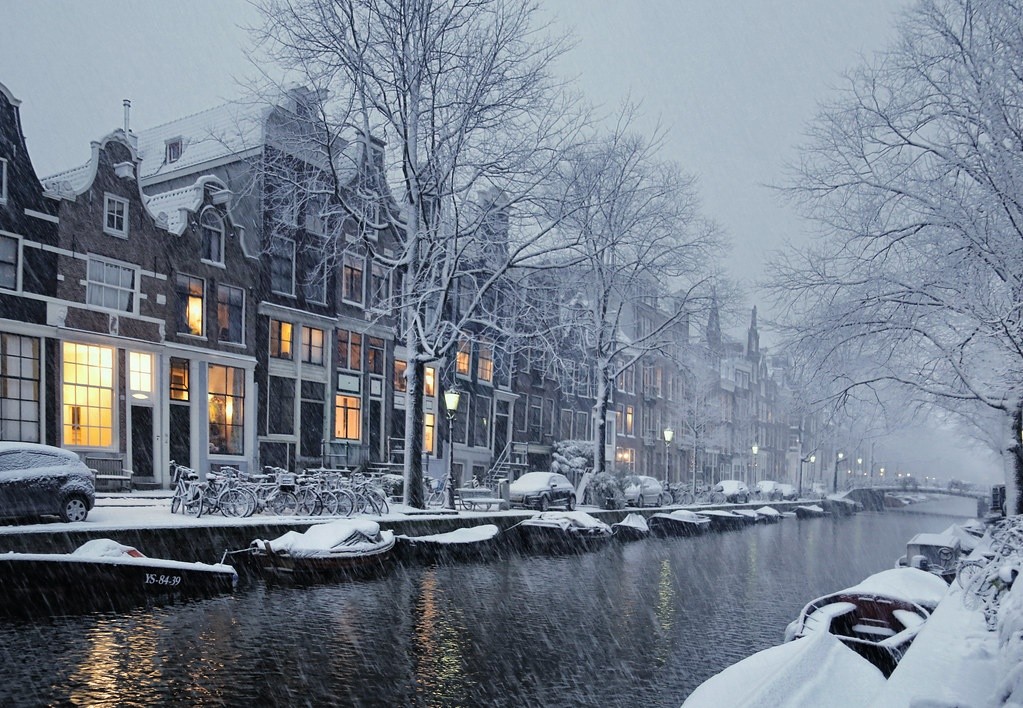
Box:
[747,488,762,503]
[956,513,1023,611]
[422,473,492,512]
[661,479,727,506]
[168,460,389,518]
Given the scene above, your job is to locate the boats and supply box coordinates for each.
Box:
[791,505,825,519]
[647,510,712,537]
[940,524,981,555]
[732,509,759,525]
[521,513,614,549]
[396,524,500,556]
[0,537,239,609]
[249,519,396,582]
[756,508,782,523]
[894,532,965,585]
[679,630,888,708]
[959,519,986,536]
[782,566,951,682]
[884,493,928,507]
[611,513,649,540]
[696,509,744,528]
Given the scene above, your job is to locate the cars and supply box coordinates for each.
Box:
[779,484,798,501]
[0,440,95,523]
[623,475,664,508]
[755,480,783,501]
[717,480,749,504]
[509,472,577,512]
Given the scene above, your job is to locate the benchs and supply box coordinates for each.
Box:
[455,488,506,512]
[85,456,135,493]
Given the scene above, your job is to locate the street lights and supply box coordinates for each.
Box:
[751,443,759,487]
[810,455,816,498]
[857,457,862,486]
[664,426,674,491]
[442,386,462,510]
[833,453,844,494]
